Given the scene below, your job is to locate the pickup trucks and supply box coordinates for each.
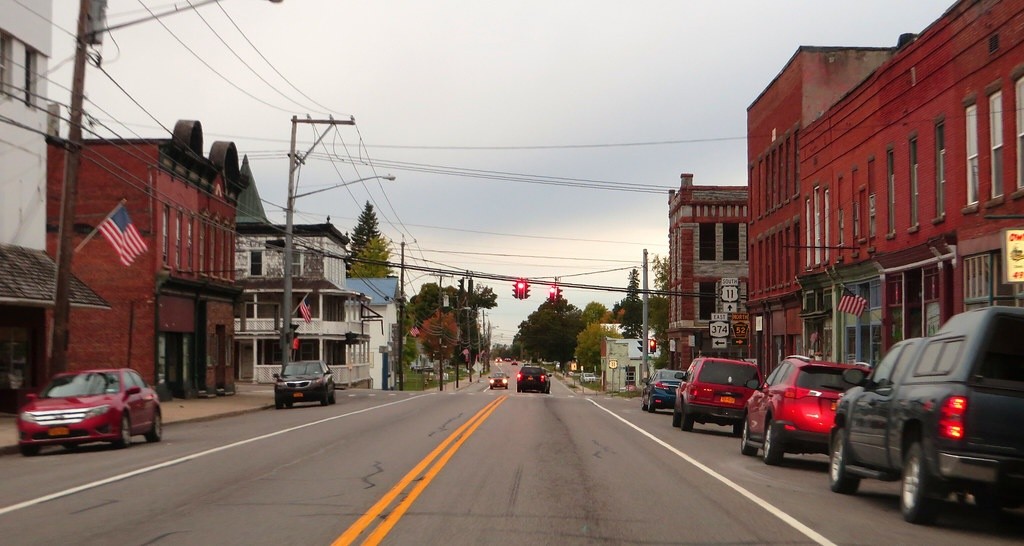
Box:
[825,303,1024,532]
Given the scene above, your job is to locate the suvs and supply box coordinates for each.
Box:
[672,355,765,436]
[516,365,552,395]
[740,353,874,464]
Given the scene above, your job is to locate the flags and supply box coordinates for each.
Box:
[97,205,147,268]
[838,286,868,319]
[463,349,469,356]
[409,324,419,338]
[299,297,312,324]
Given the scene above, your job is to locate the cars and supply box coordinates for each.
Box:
[579,373,595,383]
[495,356,503,362]
[272,360,336,409]
[512,360,518,365]
[505,358,511,362]
[16,367,164,455]
[641,369,686,412]
[488,373,510,390]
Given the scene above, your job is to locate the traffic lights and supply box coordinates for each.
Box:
[517,280,525,299]
[637,339,644,352]
[556,286,562,303]
[512,282,518,299]
[290,322,300,339]
[523,281,531,299]
[549,287,555,302]
[650,339,656,353]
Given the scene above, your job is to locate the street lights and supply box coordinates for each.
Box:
[454,305,473,390]
[40,0,284,381]
[397,272,437,391]
[279,174,397,368]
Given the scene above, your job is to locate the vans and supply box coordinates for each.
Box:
[521,363,531,367]
